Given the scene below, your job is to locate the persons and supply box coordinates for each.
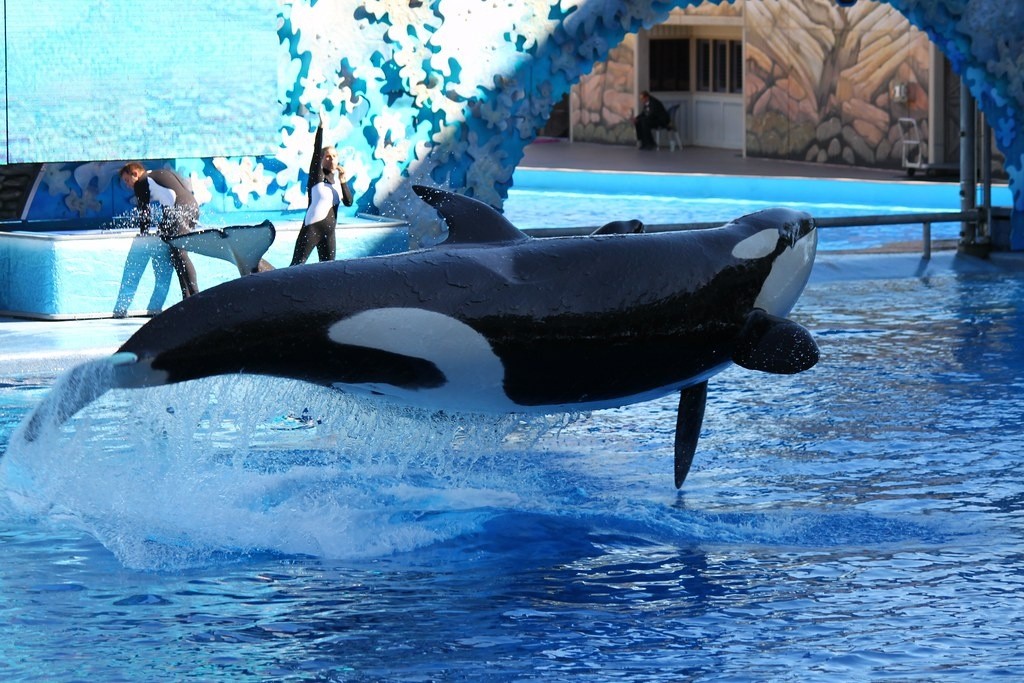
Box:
[632,91,670,149]
[290,108,353,265]
[120,163,199,300]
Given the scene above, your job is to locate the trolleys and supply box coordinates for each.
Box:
[896,116,960,176]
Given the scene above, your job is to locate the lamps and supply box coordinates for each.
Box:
[892,81,907,104]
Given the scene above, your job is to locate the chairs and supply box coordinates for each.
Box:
[656,104,683,153]
